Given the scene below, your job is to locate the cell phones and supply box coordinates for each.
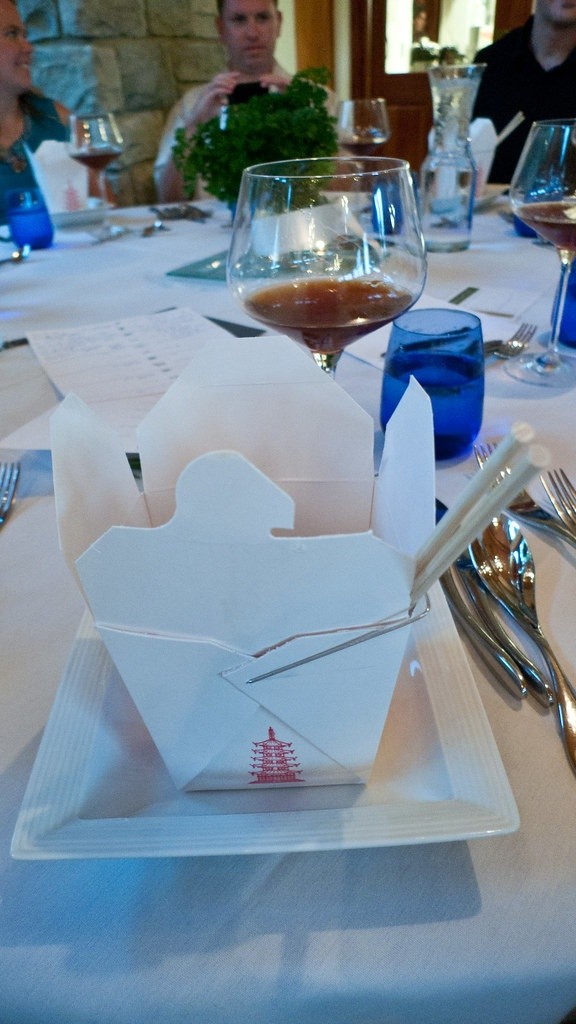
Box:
[227,81,269,105]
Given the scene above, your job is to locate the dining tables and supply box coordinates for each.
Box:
[0,190,575,1024]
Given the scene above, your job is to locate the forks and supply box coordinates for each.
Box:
[484,323,537,372]
[539,468,576,536]
[476,441,576,550]
[0,463,21,525]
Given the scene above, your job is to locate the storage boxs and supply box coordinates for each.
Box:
[428,118,500,195]
[22,140,91,214]
[51,329,436,793]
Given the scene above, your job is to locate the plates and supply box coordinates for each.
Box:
[9,577,521,859]
[33,194,114,229]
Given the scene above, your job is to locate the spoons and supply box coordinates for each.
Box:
[470,514,576,775]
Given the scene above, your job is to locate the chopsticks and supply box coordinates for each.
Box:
[408,420,550,609]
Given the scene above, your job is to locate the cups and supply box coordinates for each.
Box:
[0,188,54,251]
[550,252,576,349]
[377,309,485,459]
[372,172,419,245]
[417,63,485,251]
[516,176,564,240]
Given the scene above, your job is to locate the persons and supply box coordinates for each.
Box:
[413,9,430,38]
[155,0,355,207]
[469,2,576,185]
[0,1,112,226]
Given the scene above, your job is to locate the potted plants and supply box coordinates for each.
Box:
[171,68,384,264]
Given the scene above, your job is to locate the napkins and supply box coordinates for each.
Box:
[27,306,237,454]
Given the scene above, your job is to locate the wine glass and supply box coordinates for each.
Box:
[228,158,426,378]
[506,118,576,390]
[66,114,125,240]
[337,98,393,189]
